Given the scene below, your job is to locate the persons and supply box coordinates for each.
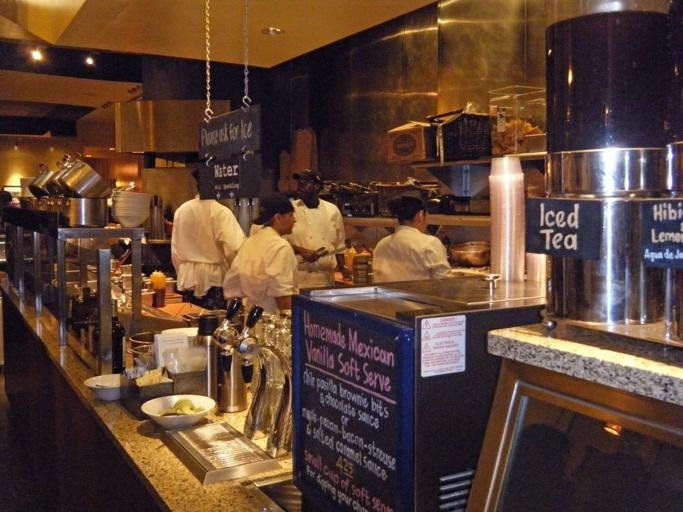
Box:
[221,191,301,318]
[369,193,451,283]
[279,166,354,289]
[0,190,21,235]
[170,167,248,309]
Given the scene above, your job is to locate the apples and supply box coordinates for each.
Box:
[174,399,194,411]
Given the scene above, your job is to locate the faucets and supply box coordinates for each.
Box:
[215,296,265,388]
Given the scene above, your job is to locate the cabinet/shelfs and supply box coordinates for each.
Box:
[341,152,548,227]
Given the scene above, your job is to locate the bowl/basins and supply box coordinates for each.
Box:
[140,392,216,429]
[83,373,121,402]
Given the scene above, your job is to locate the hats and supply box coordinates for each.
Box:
[292,169,325,188]
[251,193,296,225]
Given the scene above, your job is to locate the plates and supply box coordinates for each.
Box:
[111,191,152,227]
